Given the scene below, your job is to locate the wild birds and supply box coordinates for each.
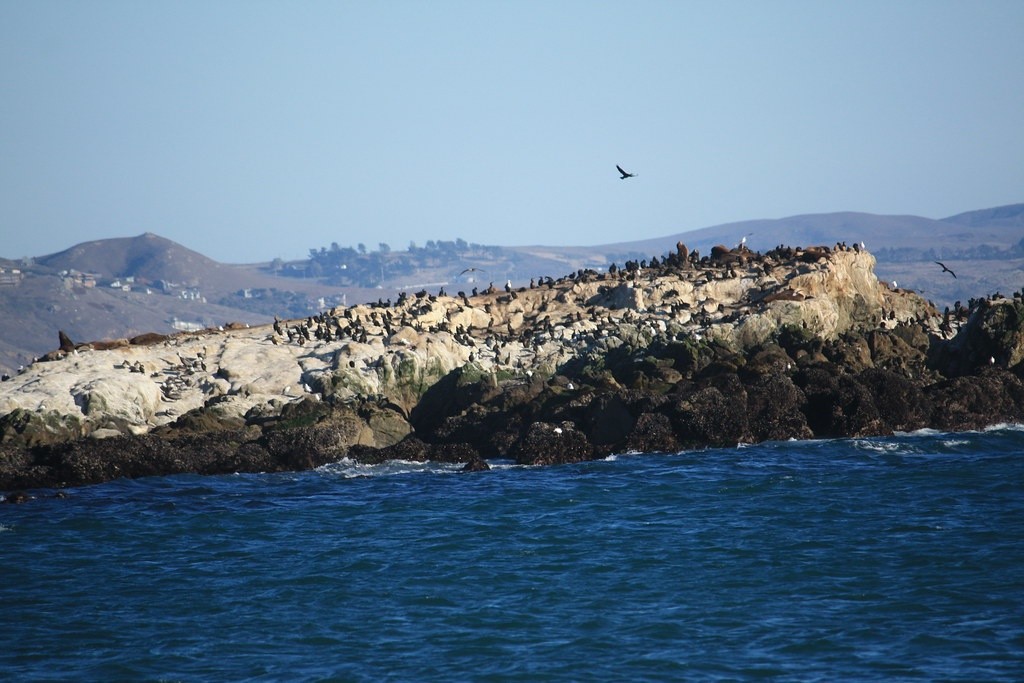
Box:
[935,262,958,279]
[736,232,864,309]
[771,281,1024,370]
[617,165,638,179]
[285,267,533,372]
[1,318,293,419]
[529,252,739,345]
